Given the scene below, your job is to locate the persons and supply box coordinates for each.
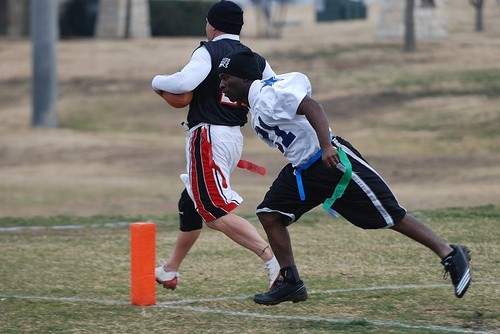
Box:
[152,0,283,292]
[219,50,472,307]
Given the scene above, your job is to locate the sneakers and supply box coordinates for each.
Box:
[264,254,281,290]
[440,244,471,298]
[155,265,178,290]
[253,281,307,303]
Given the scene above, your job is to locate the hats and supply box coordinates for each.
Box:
[208,0,243,34]
[218,52,265,81]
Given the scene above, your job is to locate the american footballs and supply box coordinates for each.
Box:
[162,90,193,108]
